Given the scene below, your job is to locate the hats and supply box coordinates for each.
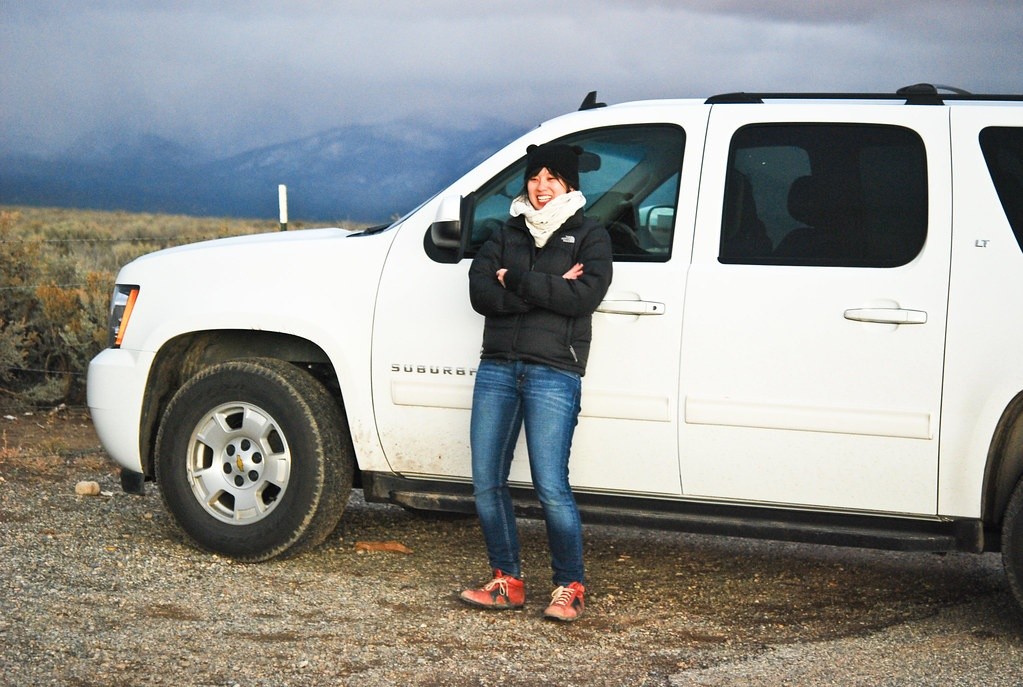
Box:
[524,143,583,182]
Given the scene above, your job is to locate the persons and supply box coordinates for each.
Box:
[461,144,612,622]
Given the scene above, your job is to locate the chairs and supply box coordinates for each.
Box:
[767,178,836,264]
[833,173,900,261]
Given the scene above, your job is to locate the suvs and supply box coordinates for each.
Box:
[84,81,1023,614]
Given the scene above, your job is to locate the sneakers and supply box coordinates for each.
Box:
[542,581,585,621]
[460,568,525,609]
